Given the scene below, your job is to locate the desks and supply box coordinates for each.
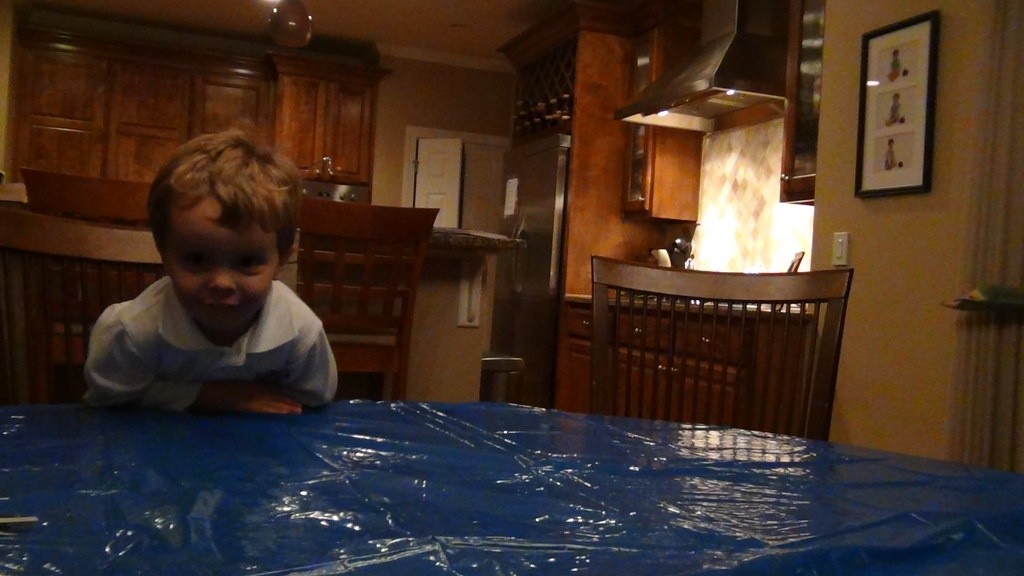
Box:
[0,401,1024,576]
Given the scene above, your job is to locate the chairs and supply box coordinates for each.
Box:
[588,255,855,441]
[296,193,441,401]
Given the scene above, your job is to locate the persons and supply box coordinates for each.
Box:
[884,138,897,170]
[887,49,901,80]
[885,94,902,127]
[83,129,339,411]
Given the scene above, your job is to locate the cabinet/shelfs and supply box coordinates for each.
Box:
[554,294,813,434]
[621,24,703,222]
[778,0,826,206]
[6,0,383,187]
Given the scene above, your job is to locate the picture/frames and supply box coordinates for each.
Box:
[853,9,941,199]
